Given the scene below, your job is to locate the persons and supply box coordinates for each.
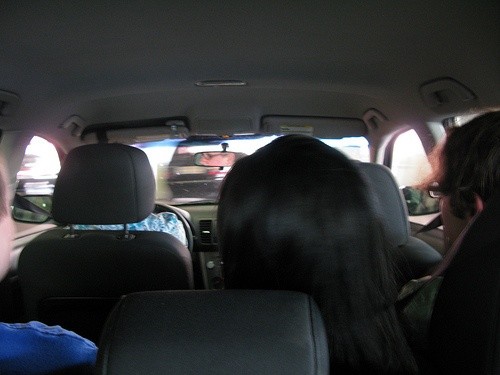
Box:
[73,141,190,252]
[213,132,420,374]
[198,150,236,167]
[1,152,104,375]
[398,109,499,358]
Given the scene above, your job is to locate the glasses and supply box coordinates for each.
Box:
[200,152,225,160]
[426,180,469,201]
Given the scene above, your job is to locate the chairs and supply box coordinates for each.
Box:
[97,289,330,375]
[19,144,201,349]
[356,162,445,301]
